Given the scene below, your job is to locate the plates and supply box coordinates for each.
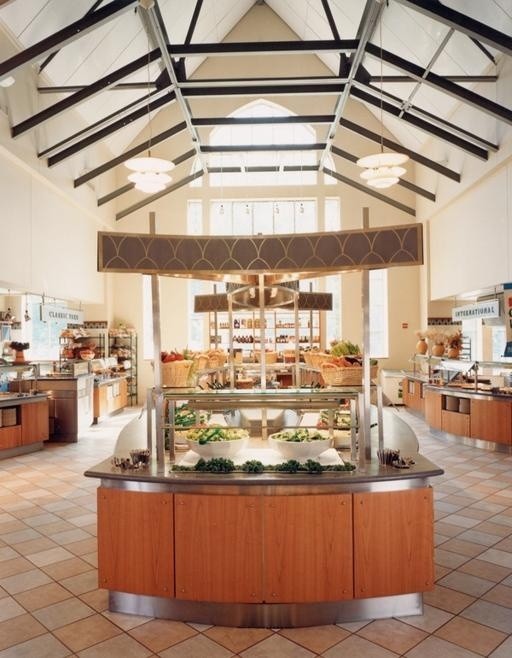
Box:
[408,380,414,394]
[2,407,17,426]
[457,398,469,414]
[444,395,458,412]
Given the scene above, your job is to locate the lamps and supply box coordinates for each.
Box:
[123,0,177,197]
[355,1,410,193]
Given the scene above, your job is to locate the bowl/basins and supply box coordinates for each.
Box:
[80,350,95,361]
[269,434,333,460]
[185,433,249,459]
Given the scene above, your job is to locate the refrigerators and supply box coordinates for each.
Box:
[379,369,421,406]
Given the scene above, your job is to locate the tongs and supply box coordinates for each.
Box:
[197,425,221,444]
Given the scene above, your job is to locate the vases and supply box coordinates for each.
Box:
[432,343,445,355]
[416,339,428,354]
[447,347,459,358]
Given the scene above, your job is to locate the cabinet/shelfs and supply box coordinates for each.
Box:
[60,332,138,407]
